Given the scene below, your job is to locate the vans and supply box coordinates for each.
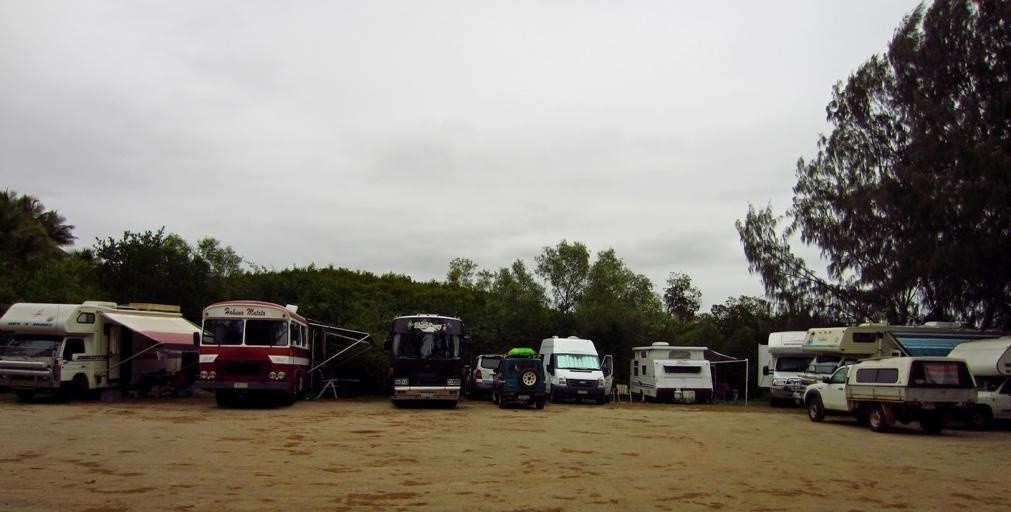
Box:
[539,336,615,405]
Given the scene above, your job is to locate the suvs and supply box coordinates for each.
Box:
[491,356,546,410]
[469,354,503,394]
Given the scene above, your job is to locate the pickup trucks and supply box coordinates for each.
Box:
[804,357,978,434]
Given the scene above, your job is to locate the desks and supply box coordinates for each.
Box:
[316,378,361,400]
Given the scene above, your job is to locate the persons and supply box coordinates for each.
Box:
[601,358,609,403]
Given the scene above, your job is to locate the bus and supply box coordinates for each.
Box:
[192,303,313,408]
[384,313,470,408]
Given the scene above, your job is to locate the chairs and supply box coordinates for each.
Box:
[615,383,632,403]
